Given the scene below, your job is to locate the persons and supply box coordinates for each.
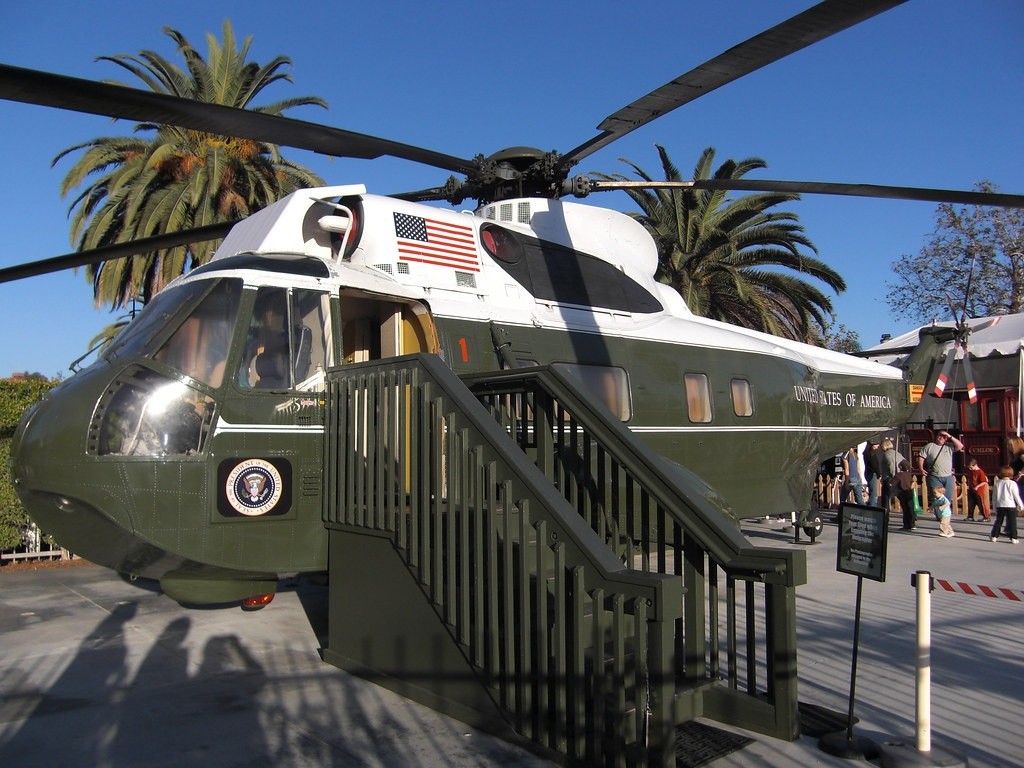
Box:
[966,458,991,522]
[991,437,1024,543]
[919,430,964,538]
[840,439,917,532]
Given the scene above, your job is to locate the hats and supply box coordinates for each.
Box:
[867,437,880,445]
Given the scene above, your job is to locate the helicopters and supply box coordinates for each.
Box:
[0,2,1022,766]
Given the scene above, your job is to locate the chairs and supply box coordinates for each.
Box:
[255,325,312,389]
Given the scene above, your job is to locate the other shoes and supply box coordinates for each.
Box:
[915,517,918,521]
[911,526,916,530]
[940,531,954,537]
[978,518,990,522]
[777,518,785,522]
[899,527,911,532]
[990,536,997,542]
[963,518,974,521]
[1012,538,1019,544]
[829,515,840,524]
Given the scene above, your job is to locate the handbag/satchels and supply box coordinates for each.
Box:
[923,461,934,474]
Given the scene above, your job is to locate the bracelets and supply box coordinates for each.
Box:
[950,435,952,439]
